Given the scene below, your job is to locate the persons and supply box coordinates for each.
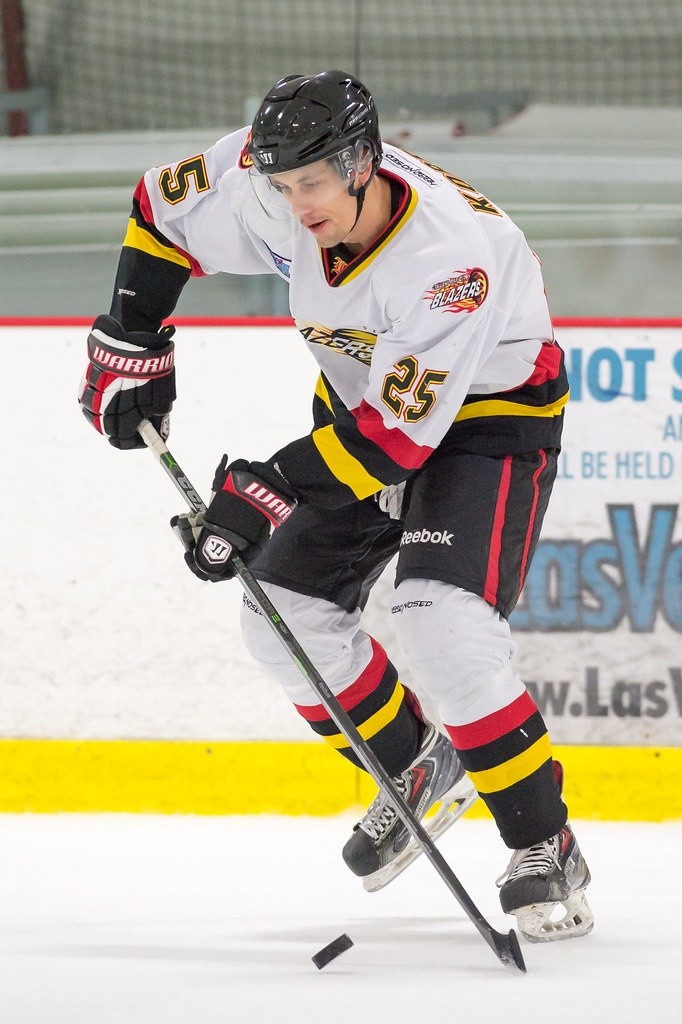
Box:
[77,71,601,942]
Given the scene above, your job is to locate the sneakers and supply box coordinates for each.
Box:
[342,684,478,893]
[499,760,595,943]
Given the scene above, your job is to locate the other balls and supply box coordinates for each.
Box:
[311,932,355,969]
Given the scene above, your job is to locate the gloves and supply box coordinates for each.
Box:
[78,314,176,450]
[184,454,297,583]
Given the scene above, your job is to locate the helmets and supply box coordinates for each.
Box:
[248,69,383,175]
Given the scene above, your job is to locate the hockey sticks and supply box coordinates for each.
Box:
[137,417,533,974]
